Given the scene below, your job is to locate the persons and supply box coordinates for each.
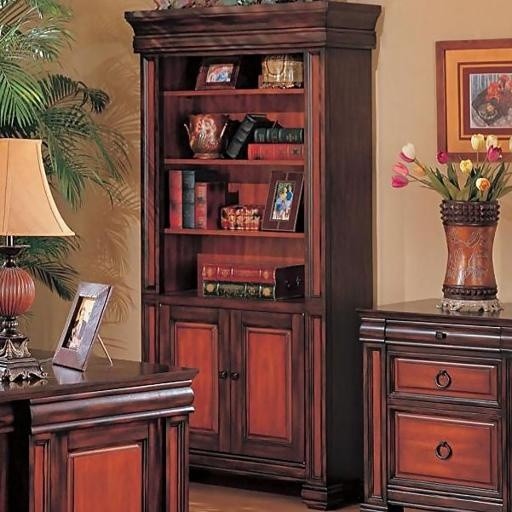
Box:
[69,306,86,345]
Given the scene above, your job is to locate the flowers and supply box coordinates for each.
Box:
[388,130,512,203]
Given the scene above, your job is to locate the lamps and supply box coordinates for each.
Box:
[0,136,77,383]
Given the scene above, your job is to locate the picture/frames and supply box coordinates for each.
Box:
[260,167,305,232]
[432,38,512,164]
[194,59,241,91]
[53,281,117,371]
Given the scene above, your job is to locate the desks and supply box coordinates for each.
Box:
[0,347,201,511]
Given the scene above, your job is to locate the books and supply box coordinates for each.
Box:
[253,126,305,144]
[168,170,184,229]
[226,113,274,159]
[247,143,305,161]
[183,167,218,229]
[194,179,227,230]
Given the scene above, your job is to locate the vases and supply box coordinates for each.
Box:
[435,198,504,313]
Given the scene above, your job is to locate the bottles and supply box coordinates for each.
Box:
[185,112,232,159]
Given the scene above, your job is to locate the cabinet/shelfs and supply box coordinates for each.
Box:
[354,291,511,511]
[122,2,384,512]
